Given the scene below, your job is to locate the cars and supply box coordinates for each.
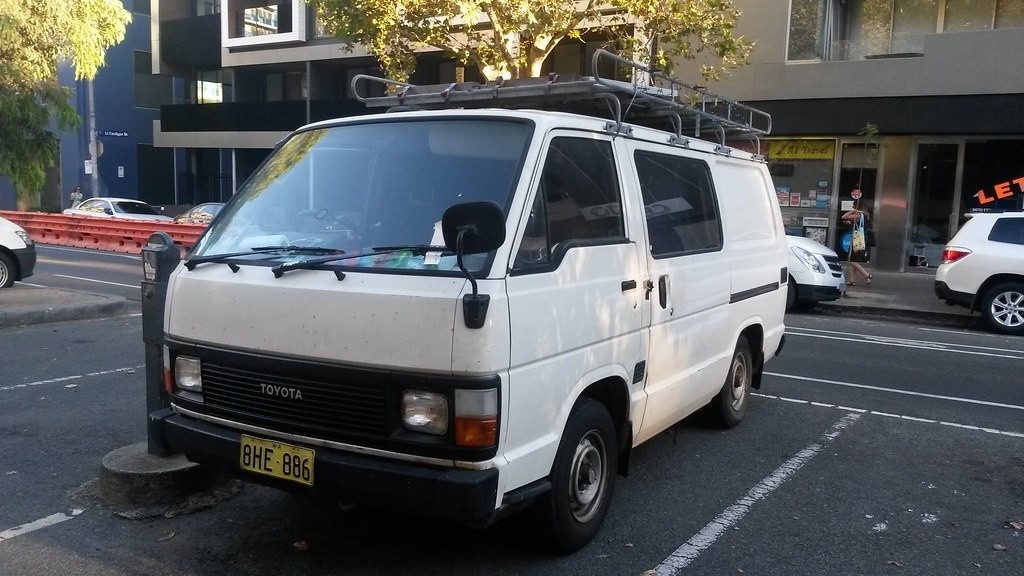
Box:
[61,198,174,221]
[784,227,846,313]
[0,216,37,290]
[172,202,227,224]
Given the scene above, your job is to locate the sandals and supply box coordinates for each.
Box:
[865,274,872,287]
[846,281,856,286]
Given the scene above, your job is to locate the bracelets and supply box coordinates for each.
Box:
[848,212,851,215]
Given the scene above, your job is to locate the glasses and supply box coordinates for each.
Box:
[77,189,80,190]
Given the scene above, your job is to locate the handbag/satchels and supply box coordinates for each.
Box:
[852,212,866,253]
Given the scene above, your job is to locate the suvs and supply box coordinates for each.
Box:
[934,207,1024,335]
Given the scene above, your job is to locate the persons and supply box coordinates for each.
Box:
[842,199,872,286]
[70,186,82,208]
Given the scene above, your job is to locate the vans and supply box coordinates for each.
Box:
[151,48,791,556]
